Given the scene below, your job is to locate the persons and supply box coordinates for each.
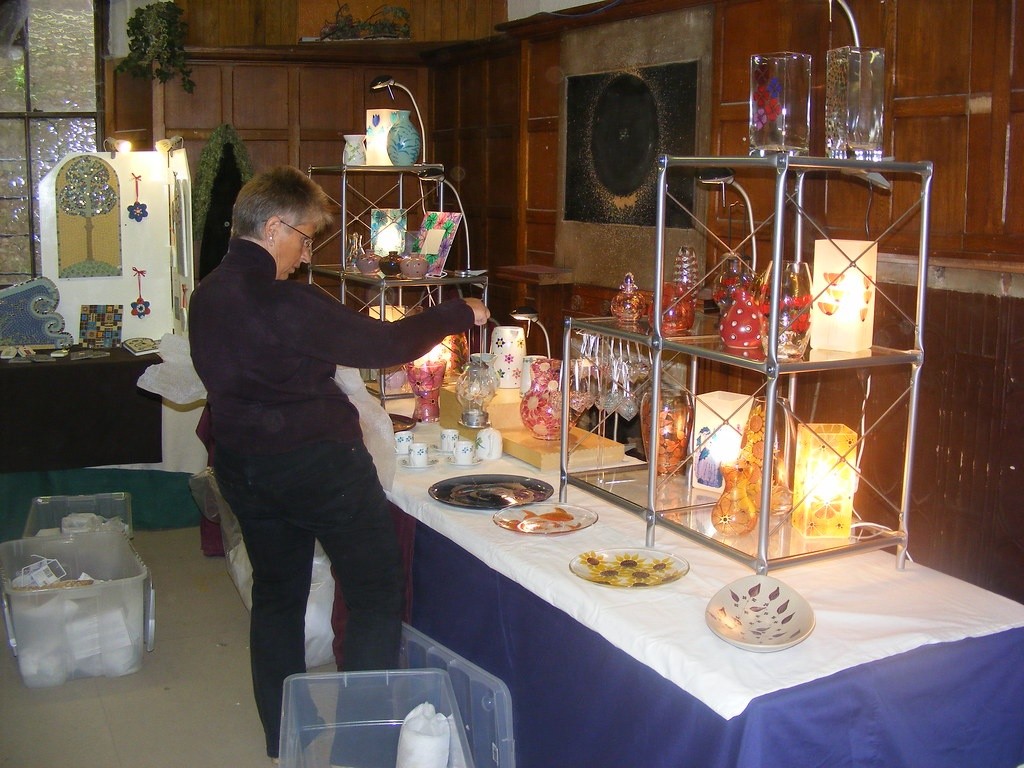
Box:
[187,166,490,768]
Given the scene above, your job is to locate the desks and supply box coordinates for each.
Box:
[0,342,209,544]
[365,383,1024,768]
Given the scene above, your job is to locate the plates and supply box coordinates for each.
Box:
[429,442,454,455]
[705,575,816,652]
[444,456,484,466]
[569,547,691,590]
[397,455,442,468]
[493,503,598,537]
[428,474,555,511]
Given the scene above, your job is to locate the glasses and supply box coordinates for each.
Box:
[280,220,314,247]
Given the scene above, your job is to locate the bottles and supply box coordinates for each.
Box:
[341,134,370,166]
[346,233,430,281]
[737,396,797,515]
[470,352,499,392]
[647,281,695,334]
[640,362,694,477]
[387,110,421,165]
[610,272,646,326]
[519,359,573,441]
[712,459,758,538]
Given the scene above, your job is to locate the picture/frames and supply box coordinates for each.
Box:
[410,211,462,277]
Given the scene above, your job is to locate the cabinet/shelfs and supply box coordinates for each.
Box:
[111,58,433,315]
[418,0,1024,603]
[559,152,934,577]
[306,162,488,409]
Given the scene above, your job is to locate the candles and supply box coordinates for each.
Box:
[370,208,407,257]
[809,238,878,353]
[790,422,858,539]
[364,108,399,167]
[691,390,755,494]
[414,330,452,386]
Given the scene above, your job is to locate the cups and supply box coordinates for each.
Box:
[748,48,812,158]
[759,260,814,360]
[475,428,503,461]
[453,440,475,464]
[825,46,885,162]
[393,431,414,454]
[408,443,429,466]
[490,326,527,389]
[519,355,547,398]
[440,429,460,452]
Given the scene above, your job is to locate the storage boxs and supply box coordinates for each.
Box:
[21,492,135,544]
[277,668,475,768]
[0,529,150,689]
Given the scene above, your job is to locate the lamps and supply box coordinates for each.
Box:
[369,74,426,165]
[509,306,551,359]
[154,135,182,155]
[697,167,757,277]
[107,136,132,154]
[418,168,471,270]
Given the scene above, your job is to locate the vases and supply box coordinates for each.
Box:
[405,357,447,424]
[519,357,573,441]
[711,456,758,538]
[639,387,694,479]
[489,325,527,389]
[386,109,422,167]
